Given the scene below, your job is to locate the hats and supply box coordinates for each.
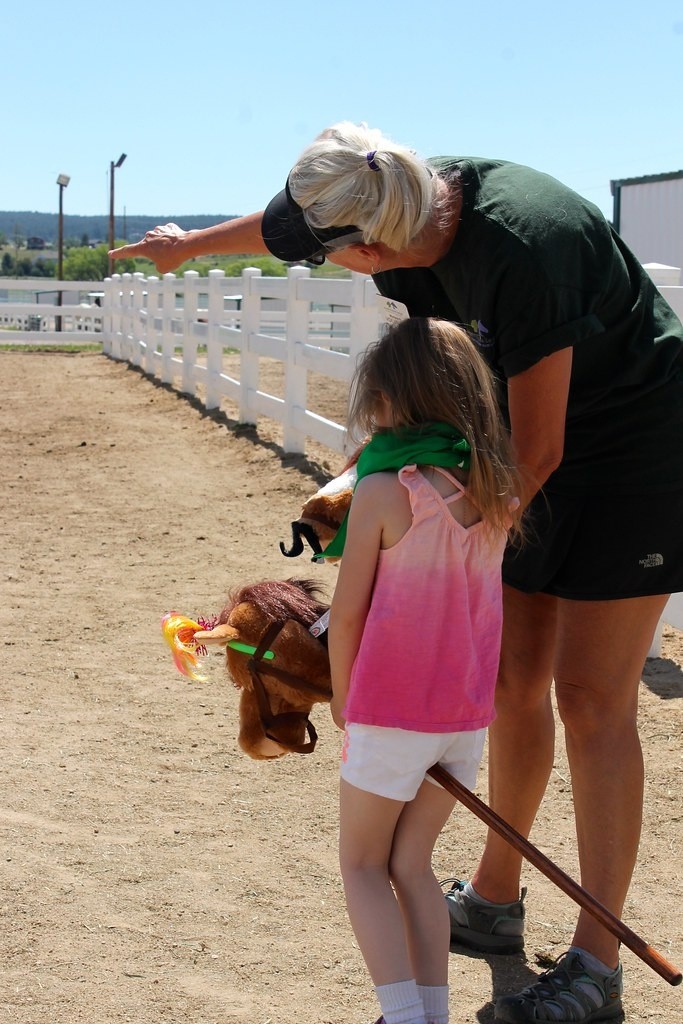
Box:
[261,171,372,262]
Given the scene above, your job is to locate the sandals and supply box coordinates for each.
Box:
[496,952,624,1022]
[438,877,528,955]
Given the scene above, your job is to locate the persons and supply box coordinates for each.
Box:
[326,317,522,1024]
[109,123,683,1024]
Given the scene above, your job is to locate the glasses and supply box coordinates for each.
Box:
[304,246,335,266]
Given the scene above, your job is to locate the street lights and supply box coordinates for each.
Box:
[110,153,128,278]
[56,174,72,329]
